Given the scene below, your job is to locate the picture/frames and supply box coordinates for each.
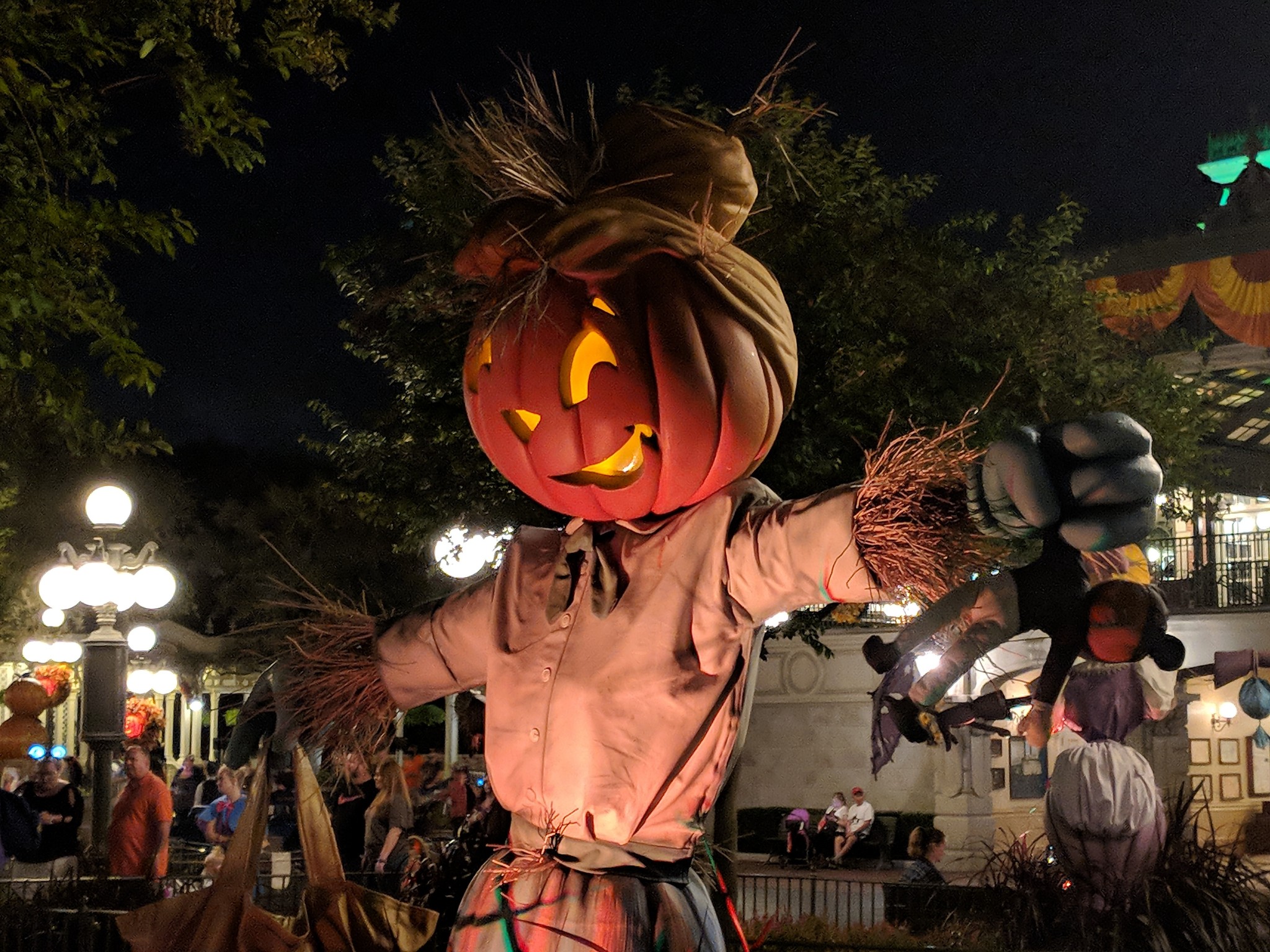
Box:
[1245,736,1270,798]
[1219,773,1242,801]
[1190,774,1213,802]
[1190,738,1211,765]
[1217,738,1241,765]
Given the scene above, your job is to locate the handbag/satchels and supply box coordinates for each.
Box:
[113,735,439,952]
[817,821,839,835]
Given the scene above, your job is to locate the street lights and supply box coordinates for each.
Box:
[35,482,175,871]
[22,638,84,759]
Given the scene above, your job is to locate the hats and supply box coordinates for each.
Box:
[852,787,862,795]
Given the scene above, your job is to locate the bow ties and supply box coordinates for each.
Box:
[215,800,235,836]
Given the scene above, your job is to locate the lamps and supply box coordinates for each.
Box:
[1208,702,1237,732]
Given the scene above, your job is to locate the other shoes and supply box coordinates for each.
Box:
[826,858,850,872]
[819,855,825,868]
[800,828,815,845]
[779,854,794,860]
[807,848,815,864]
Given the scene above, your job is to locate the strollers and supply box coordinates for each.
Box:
[778,813,817,873]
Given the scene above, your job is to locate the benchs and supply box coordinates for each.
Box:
[762,815,899,867]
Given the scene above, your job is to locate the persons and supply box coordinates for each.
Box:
[814,785,877,868]
[897,824,950,888]
[253,39,1166,952]
[0,722,503,915]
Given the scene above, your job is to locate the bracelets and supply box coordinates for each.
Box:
[377,858,387,863]
[59,813,66,822]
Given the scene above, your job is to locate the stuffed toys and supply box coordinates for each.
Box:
[863,516,1181,749]
